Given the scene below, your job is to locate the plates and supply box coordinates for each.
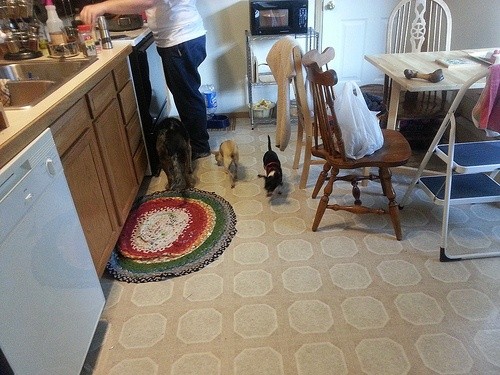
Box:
[469,50,495,65]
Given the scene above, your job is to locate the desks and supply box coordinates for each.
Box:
[363,48,500,178]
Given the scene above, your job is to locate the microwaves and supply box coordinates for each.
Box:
[249,0,309,35]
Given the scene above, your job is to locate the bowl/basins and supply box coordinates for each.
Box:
[210,113,230,128]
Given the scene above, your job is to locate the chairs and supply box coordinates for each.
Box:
[382,0,453,131]
[290,43,412,240]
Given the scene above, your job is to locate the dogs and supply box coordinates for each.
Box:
[209,139,239,189]
[155,116,196,193]
[257,134,284,197]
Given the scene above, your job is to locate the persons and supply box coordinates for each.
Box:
[77,0,210,160]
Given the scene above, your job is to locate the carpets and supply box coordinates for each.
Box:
[104,187,237,283]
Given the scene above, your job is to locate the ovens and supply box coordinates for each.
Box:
[128,25,182,177]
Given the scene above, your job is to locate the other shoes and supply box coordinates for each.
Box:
[190,150,211,161]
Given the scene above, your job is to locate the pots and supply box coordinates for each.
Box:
[106,14,143,31]
[249,103,274,119]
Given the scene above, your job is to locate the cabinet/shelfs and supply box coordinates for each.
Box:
[49,54,147,281]
[244,28,320,131]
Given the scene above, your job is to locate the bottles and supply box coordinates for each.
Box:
[491,50,500,64]
[0,0,112,57]
[201,84,216,120]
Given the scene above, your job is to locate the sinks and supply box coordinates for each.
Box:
[0,78,57,110]
[0,57,100,85]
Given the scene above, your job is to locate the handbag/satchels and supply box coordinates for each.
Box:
[334,80,384,162]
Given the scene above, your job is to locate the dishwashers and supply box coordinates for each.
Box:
[0,127,105,375]
